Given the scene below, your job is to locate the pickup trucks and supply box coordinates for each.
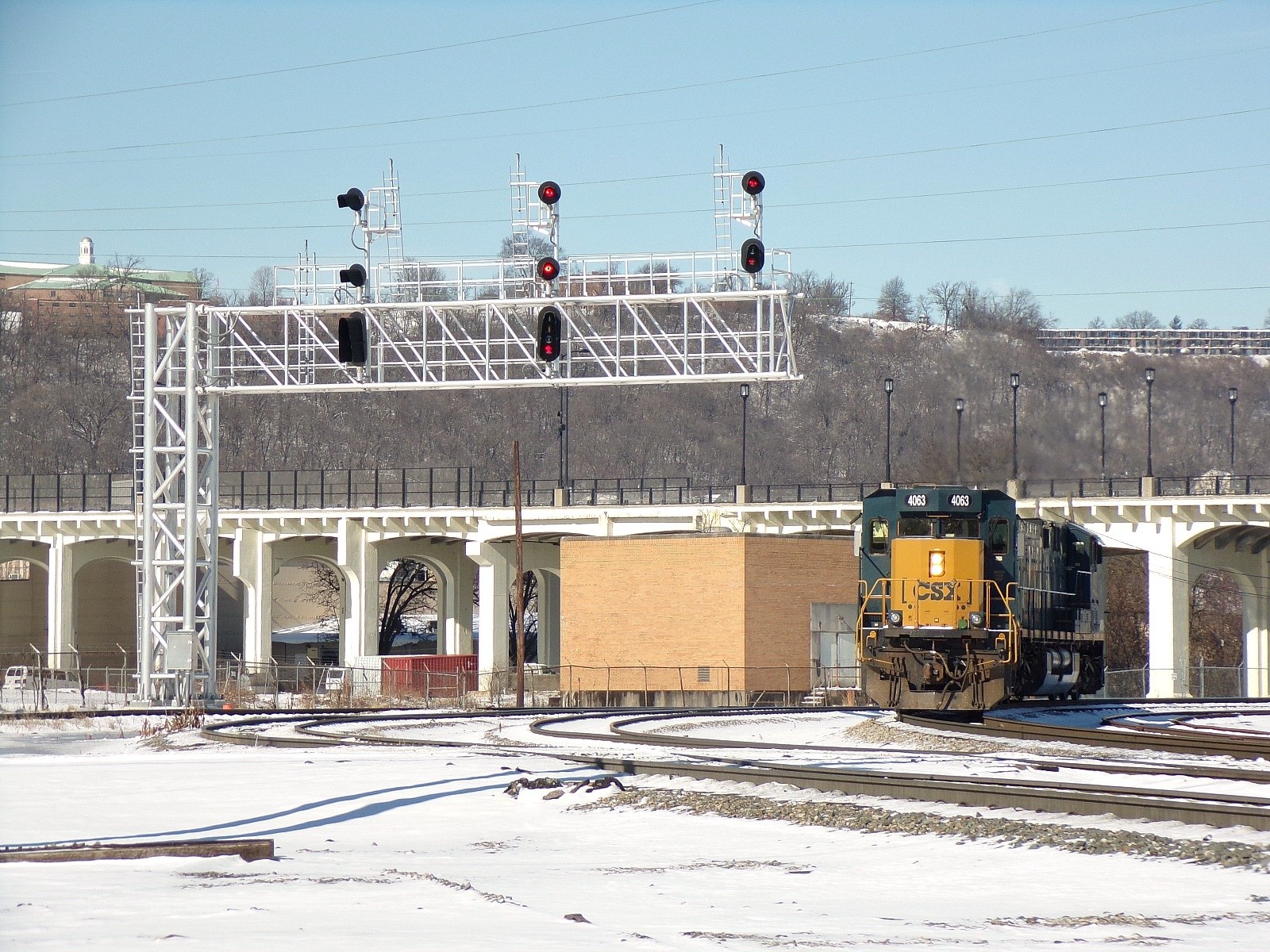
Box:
[524,663,560,675]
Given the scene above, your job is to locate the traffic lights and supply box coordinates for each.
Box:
[536,256,560,280]
[337,188,363,212]
[742,237,765,274]
[537,306,561,363]
[742,171,766,195]
[539,181,561,205]
[341,262,367,287]
[338,310,366,367]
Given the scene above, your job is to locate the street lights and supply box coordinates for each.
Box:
[157,382,181,427]
[558,349,589,489]
[884,378,894,483]
[1227,386,1237,491]
[955,397,965,485]
[1144,366,1157,477]
[741,383,749,485]
[1010,371,1020,478]
[1099,391,1108,491]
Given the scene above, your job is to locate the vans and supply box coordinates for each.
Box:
[326,667,350,692]
[2,665,84,690]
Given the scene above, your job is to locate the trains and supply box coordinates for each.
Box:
[856,486,1106,715]
